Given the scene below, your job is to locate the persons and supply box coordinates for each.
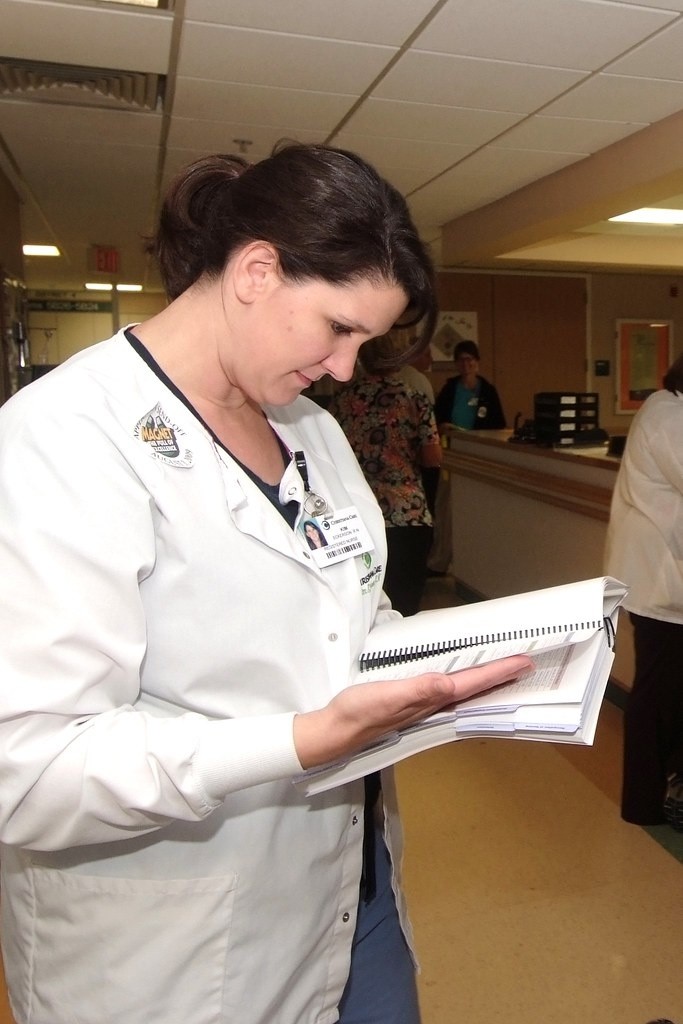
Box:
[434,339,508,430]
[304,521,328,551]
[328,332,442,615]
[602,357,683,832]
[393,337,444,571]
[0,142,531,1024]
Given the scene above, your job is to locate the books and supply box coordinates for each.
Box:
[290,576,628,797]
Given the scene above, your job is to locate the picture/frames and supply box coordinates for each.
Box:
[615,318,674,415]
